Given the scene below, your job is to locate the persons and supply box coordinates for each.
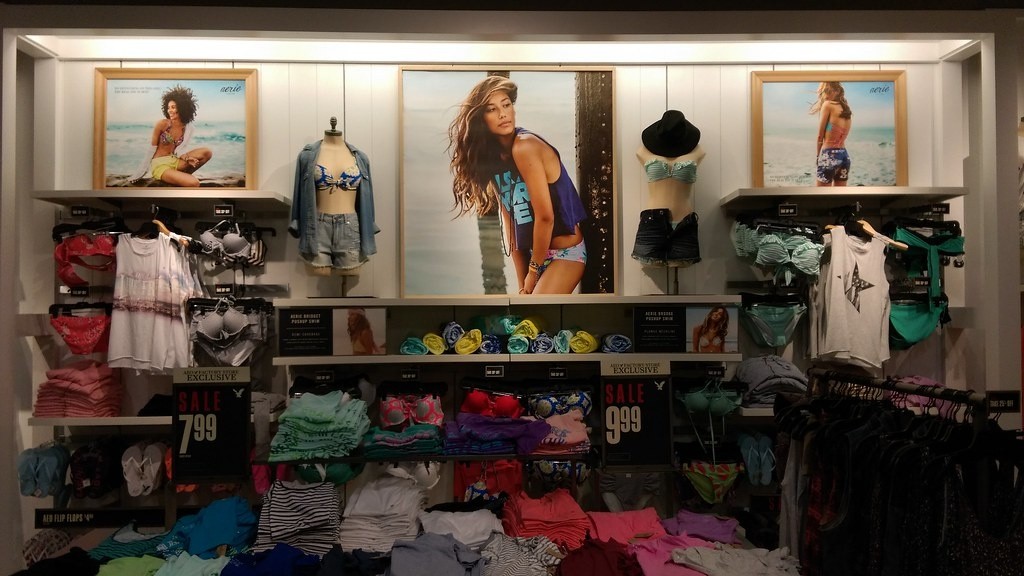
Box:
[445,76,587,295]
[692,307,729,353]
[630,109,707,268]
[127,85,213,188]
[289,116,381,276]
[809,81,852,187]
[347,309,378,354]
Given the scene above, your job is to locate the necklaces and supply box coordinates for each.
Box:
[499,158,512,257]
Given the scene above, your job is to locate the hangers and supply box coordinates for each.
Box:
[52,205,276,250]
[48,286,113,319]
[185,296,275,317]
[289,375,588,404]
[770,369,1024,482]
[740,201,966,327]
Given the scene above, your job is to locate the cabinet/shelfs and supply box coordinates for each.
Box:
[25,410,283,530]
[719,185,969,417]
[271,294,742,461]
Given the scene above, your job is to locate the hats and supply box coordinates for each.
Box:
[641,111,702,156]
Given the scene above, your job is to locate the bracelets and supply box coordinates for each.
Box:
[519,288,524,294]
[529,260,542,275]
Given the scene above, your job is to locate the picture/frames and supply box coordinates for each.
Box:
[92,67,259,190]
[750,70,908,188]
[395,65,616,296]
